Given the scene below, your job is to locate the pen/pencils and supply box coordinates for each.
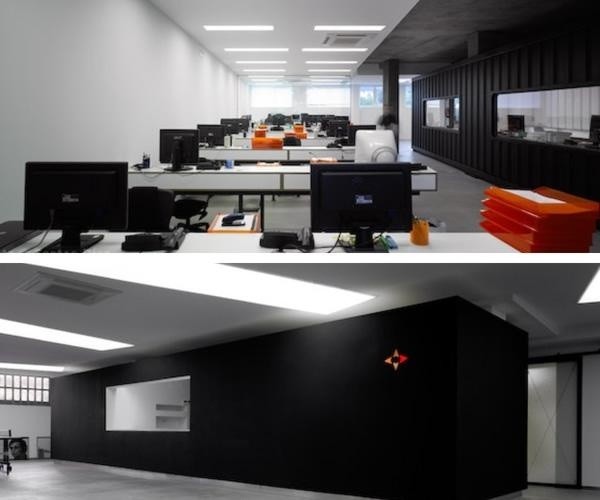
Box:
[413,213,423,225]
[143,152,151,159]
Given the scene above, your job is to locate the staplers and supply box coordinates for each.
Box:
[222,213,246,227]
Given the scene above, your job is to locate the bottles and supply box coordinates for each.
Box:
[144,153,151,169]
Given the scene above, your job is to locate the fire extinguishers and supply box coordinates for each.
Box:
[445,107,450,117]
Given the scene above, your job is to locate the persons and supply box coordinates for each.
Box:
[9,439,27,460]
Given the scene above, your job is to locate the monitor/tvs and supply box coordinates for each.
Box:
[24,162,128,253]
[159,129,199,170]
[587,115,599,148]
[310,164,413,254]
[507,114,525,132]
[199,113,376,147]
[354,130,398,163]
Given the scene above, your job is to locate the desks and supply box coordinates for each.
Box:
[0,232,600,253]
[124,108,439,233]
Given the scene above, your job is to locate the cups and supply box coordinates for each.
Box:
[410,217,430,244]
[223,149,234,168]
